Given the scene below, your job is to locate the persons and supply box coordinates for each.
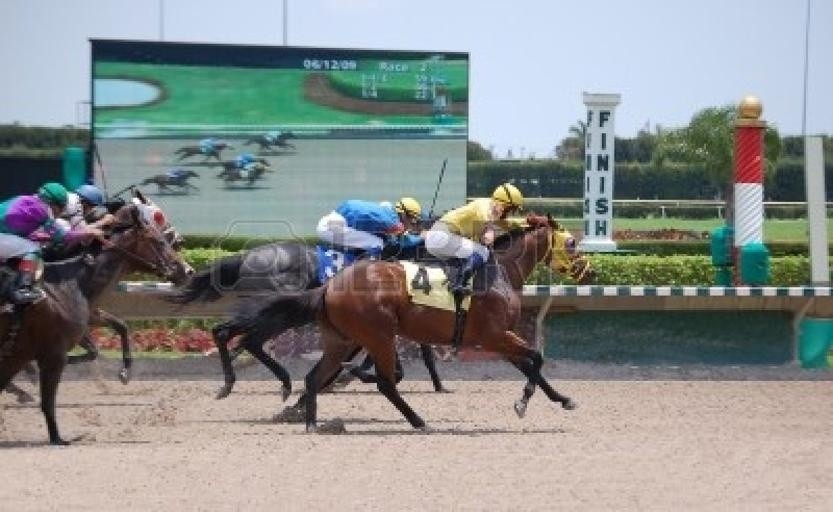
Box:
[316,179,529,292]
[1,185,119,306]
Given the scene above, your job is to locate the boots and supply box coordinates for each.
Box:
[449,252,484,294]
[12,268,42,305]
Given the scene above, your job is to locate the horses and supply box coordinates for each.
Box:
[138,167,200,195]
[419,343,447,392]
[0,184,200,446]
[158,207,456,401]
[209,158,269,177]
[233,209,601,436]
[172,138,235,163]
[242,128,299,154]
[215,163,270,186]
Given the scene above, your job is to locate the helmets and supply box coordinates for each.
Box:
[491,181,524,216]
[75,184,104,206]
[37,181,71,204]
[395,197,423,221]
